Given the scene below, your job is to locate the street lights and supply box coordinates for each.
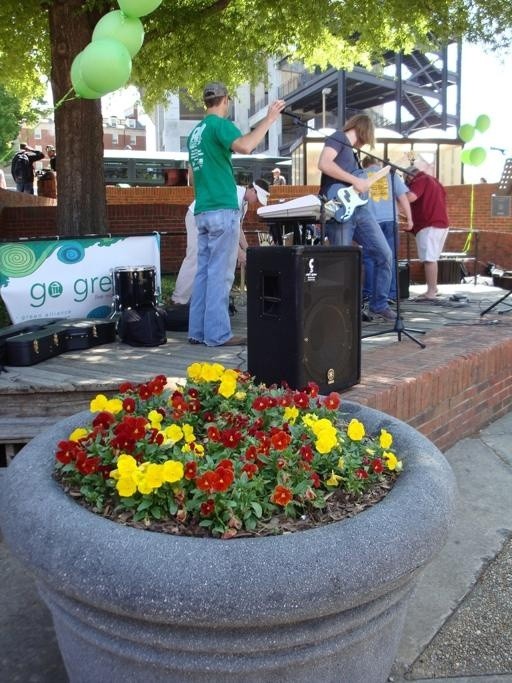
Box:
[321,88,333,129]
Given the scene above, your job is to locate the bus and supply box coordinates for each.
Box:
[103,150,294,185]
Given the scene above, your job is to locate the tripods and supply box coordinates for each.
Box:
[361,173,427,355]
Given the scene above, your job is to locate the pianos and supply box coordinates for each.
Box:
[256,194,322,222]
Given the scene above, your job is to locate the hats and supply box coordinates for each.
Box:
[252,182,269,205]
[272,168,280,173]
[203,83,233,102]
[403,165,419,186]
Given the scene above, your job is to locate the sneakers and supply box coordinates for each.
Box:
[375,304,400,320]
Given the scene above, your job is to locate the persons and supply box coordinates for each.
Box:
[186,82,286,347]
[358,156,414,304]
[46,145,57,172]
[11,143,45,195]
[170,179,271,310]
[317,114,404,323]
[399,166,450,300]
[271,168,286,185]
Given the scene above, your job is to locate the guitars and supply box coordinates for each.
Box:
[326,150,417,224]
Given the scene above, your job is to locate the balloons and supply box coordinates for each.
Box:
[459,113,490,165]
[80,37,133,95]
[117,0,162,18]
[92,10,145,59]
[70,50,103,100]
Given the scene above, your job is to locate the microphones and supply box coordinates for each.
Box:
[281,108,302,120]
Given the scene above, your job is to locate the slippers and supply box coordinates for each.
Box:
[415,290,442,301]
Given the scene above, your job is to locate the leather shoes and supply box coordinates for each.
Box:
[220,336,247,346]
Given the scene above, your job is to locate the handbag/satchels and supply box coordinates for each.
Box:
[117,306,171,349]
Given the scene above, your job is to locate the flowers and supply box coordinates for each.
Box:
[52,359,404,540]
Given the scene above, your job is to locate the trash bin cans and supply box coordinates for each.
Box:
[37,169,57,199]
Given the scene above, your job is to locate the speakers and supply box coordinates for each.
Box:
[245,243,363,396]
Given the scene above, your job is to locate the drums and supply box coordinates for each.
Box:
[110,266,158,316]
